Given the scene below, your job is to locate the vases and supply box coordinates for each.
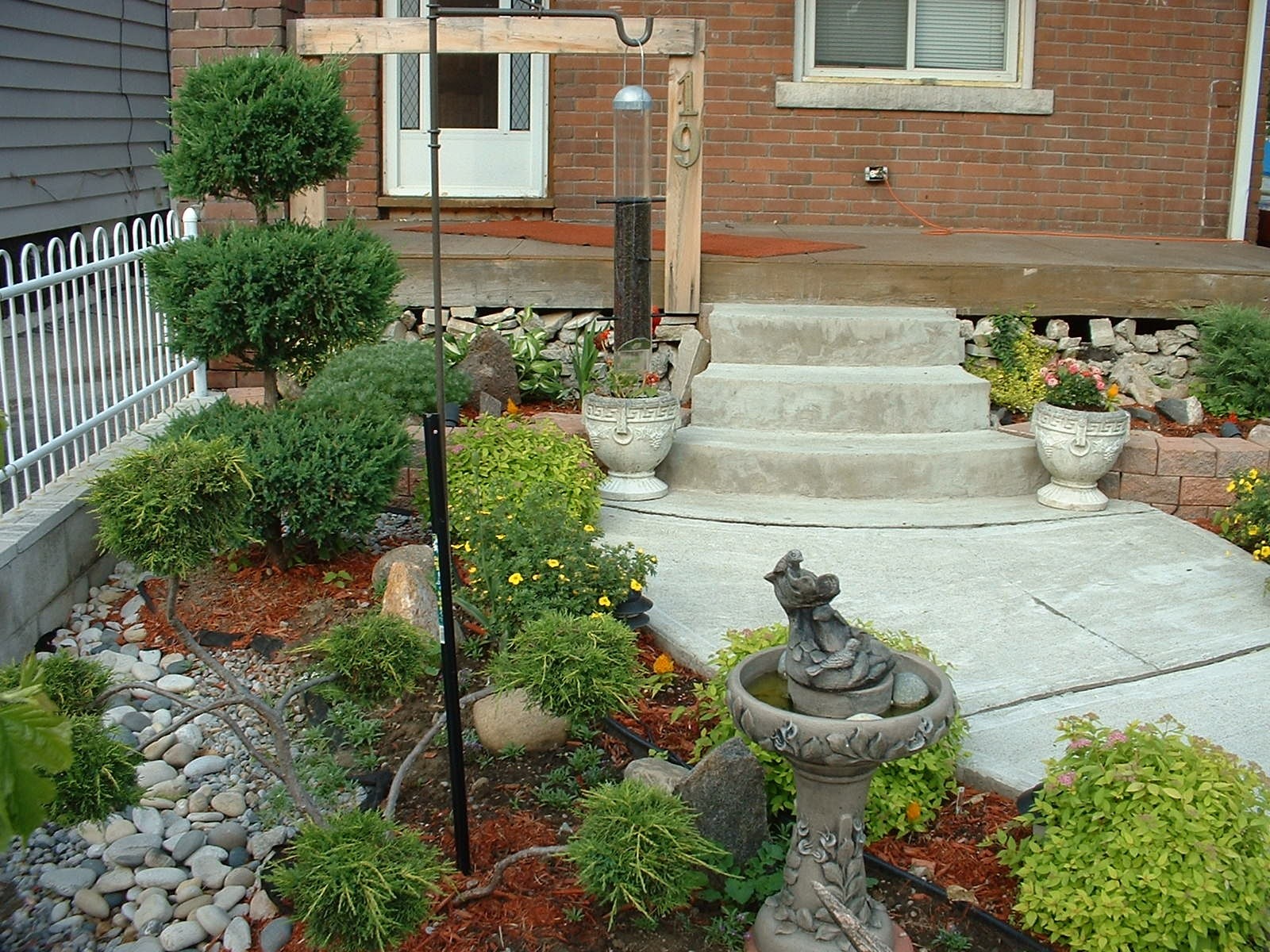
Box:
[582,390,680,501]
[1032,400,1131,510]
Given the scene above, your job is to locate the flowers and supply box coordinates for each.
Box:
[591,327,658,401]
[1039,356,1122,413]
